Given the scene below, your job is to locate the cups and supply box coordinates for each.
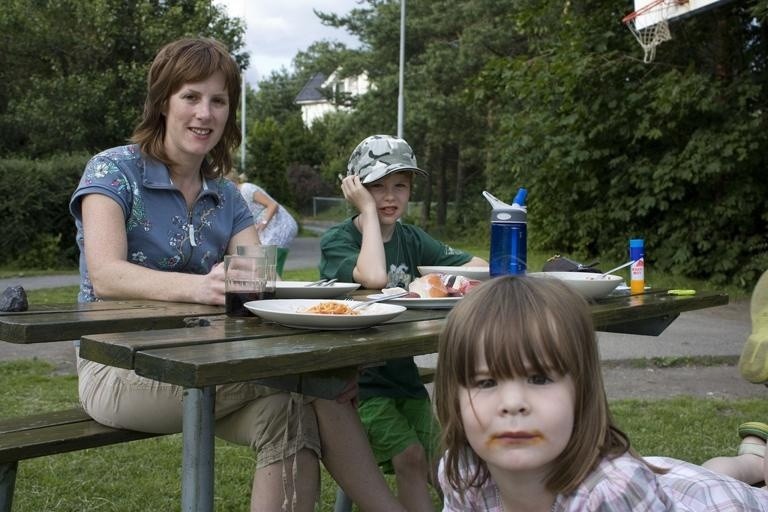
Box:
[223,243,278,319]
[482,187,528,277]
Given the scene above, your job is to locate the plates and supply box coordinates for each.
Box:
[266,280,361,299]
[243,298,407,331]
[366,292,465,309]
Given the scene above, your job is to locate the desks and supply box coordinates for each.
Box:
[2,264,728,510]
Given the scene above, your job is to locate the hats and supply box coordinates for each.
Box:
[346,134,429,185]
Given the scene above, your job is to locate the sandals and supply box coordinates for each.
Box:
[738,422,768,459]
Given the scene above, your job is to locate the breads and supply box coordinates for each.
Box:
[409,273,446,299]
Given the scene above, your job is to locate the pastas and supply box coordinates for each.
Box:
[305,303,356,316]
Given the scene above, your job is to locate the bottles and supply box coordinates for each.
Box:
[629,239,645,295]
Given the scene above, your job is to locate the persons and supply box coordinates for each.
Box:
[438,275,768,512]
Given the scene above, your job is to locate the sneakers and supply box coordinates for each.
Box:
[738,269,768,384]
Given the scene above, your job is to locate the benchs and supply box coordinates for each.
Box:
[1,366,436,512]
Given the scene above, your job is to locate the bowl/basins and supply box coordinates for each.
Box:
[417,265,490,281]
[526,271,624,301]
